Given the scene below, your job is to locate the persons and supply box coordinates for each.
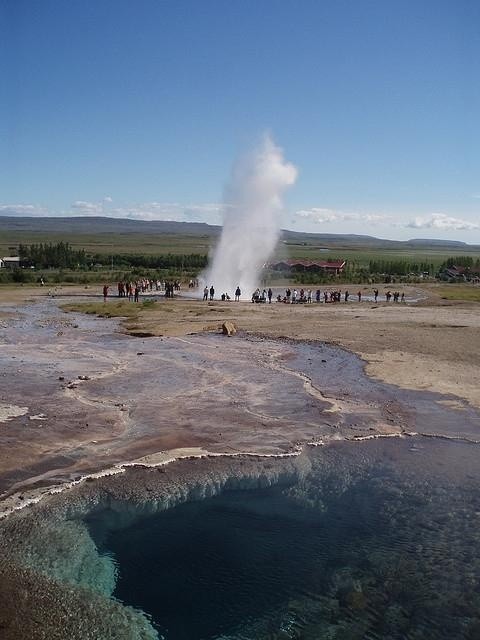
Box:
[345,290,349,302]
[374,289,378,303]
[202,286,342,304]
[102,285,109,301]
[187,278,198,288]
[116,278,181,303]
[357,291,362,302]
[385,290,405,303]
[40,275,44,286]
